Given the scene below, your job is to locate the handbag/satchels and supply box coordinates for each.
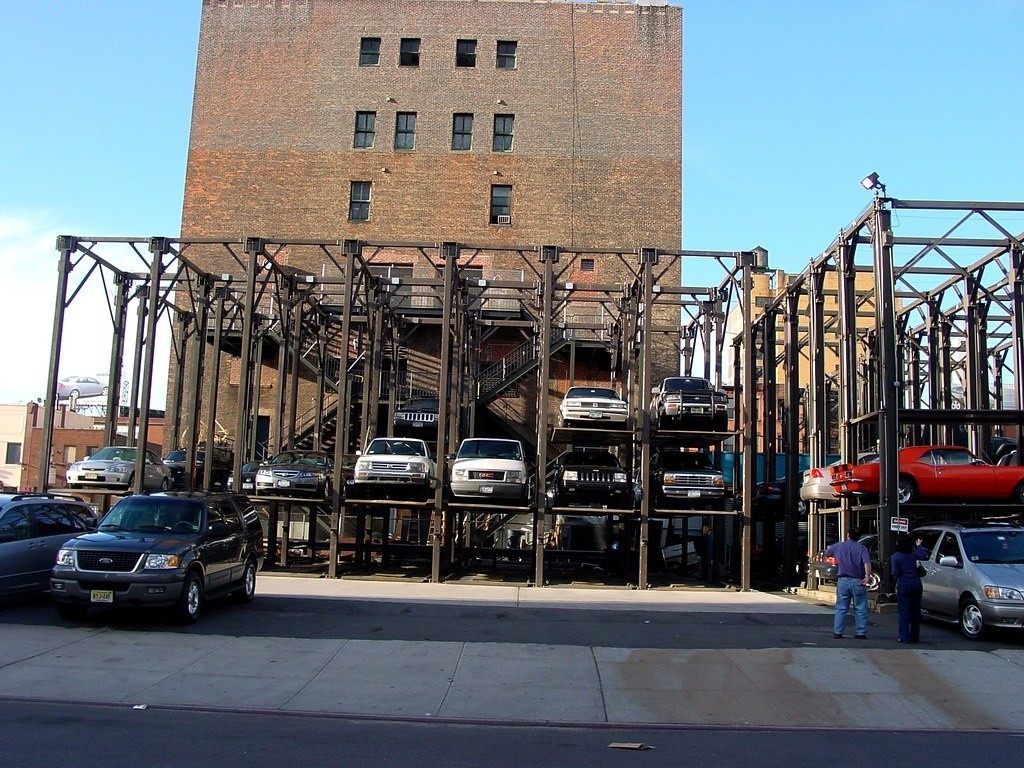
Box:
[912,550,926,577]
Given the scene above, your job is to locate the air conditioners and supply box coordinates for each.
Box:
[498,215,511,223]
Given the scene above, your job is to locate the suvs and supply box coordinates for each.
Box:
[635,448,725,510]
[0,490,99,602]
[649,376,729,429]
[354,438,434,496]
[551,450,629,506]
[905,521,1024,641]
[50,490,265,625]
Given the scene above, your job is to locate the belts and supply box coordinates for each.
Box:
[838,575,857,578]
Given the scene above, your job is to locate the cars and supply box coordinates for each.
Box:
[66,446,173,492]
[812,533,880,593]
[756,471,810,518]
[829,435,1024,506]
[57,376,109,400]
[559,385,629,428]
[800,452,878,505]
[164,449,231,490]
[227,459,262,493]
[255,450,359,498]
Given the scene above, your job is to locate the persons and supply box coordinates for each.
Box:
[697,527,713,579]
[824,527,871,640]
[891,534,930,643]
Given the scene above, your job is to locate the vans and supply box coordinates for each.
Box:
[448,438,528,501]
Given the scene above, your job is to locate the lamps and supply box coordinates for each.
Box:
[860,171,886,198]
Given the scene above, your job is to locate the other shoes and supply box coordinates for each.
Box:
[897,637,910,644]
[833,633,842,639]
[909,637,919,643]
[855,634,867,639]
